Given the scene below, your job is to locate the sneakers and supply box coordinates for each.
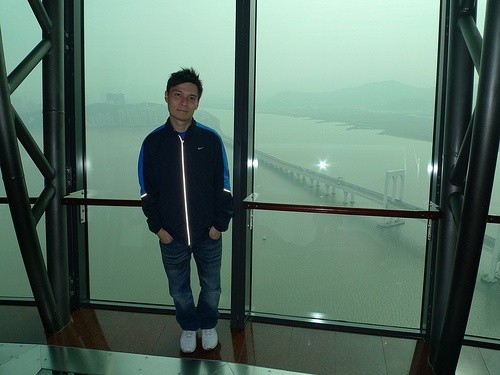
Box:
[180,331,196,352]
[201,329,217,349]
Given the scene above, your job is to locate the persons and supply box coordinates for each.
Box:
[138,67,233,352]
